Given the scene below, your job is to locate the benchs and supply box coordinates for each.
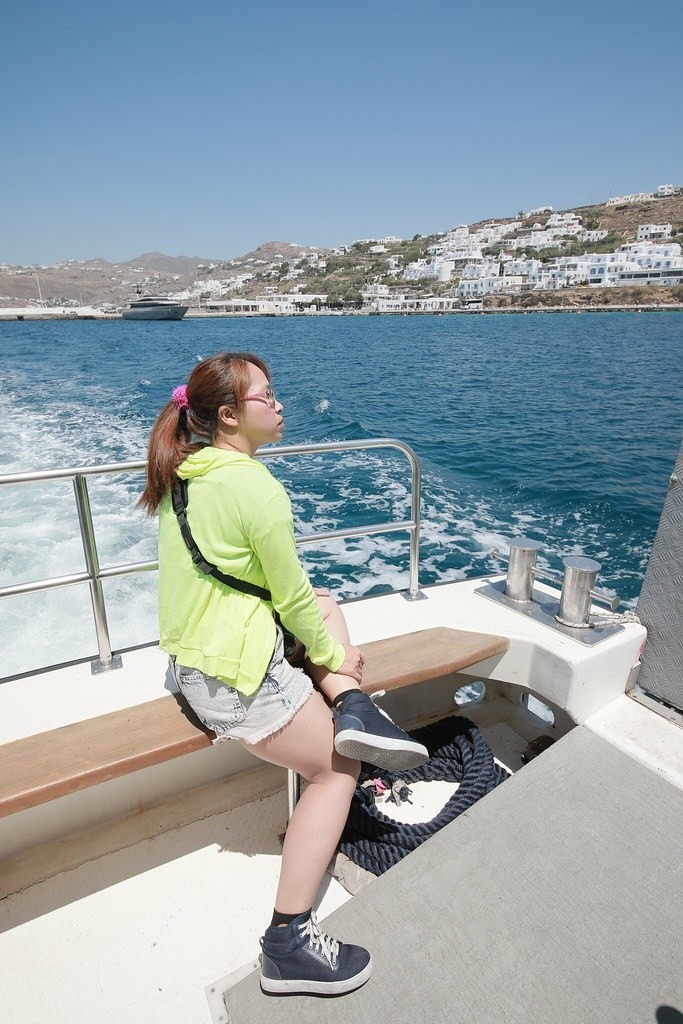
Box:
[1,628,510,819]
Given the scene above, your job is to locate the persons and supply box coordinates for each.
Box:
[129,352,429,994]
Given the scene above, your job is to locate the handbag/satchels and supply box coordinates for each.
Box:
[273,607,296,657]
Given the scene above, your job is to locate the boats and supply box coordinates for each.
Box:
[121,283,190,321]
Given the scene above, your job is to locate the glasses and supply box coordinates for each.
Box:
[229,387,277,411]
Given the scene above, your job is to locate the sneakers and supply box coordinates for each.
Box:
[332,689,428,772]
[259,906,372,995]
